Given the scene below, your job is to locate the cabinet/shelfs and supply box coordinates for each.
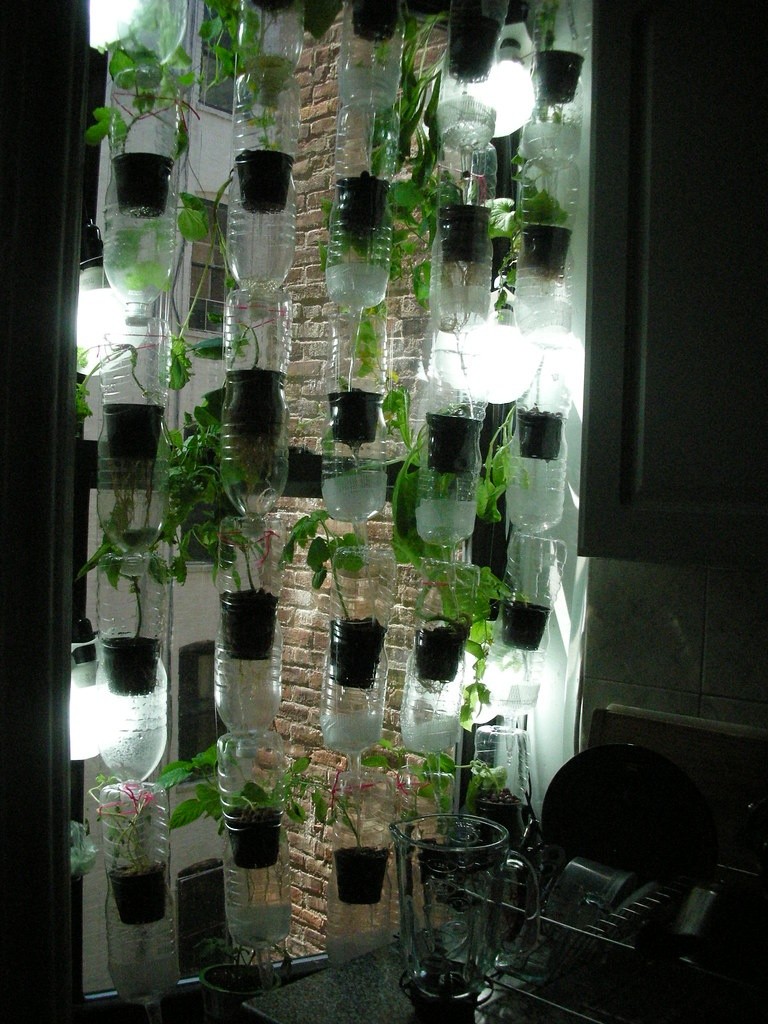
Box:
[578,0,768,572]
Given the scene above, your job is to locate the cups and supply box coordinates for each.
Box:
[390,814,538,998]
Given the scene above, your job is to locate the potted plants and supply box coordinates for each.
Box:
[72,0,588,1024]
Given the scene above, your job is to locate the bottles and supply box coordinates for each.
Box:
[98,2,591,1005]
